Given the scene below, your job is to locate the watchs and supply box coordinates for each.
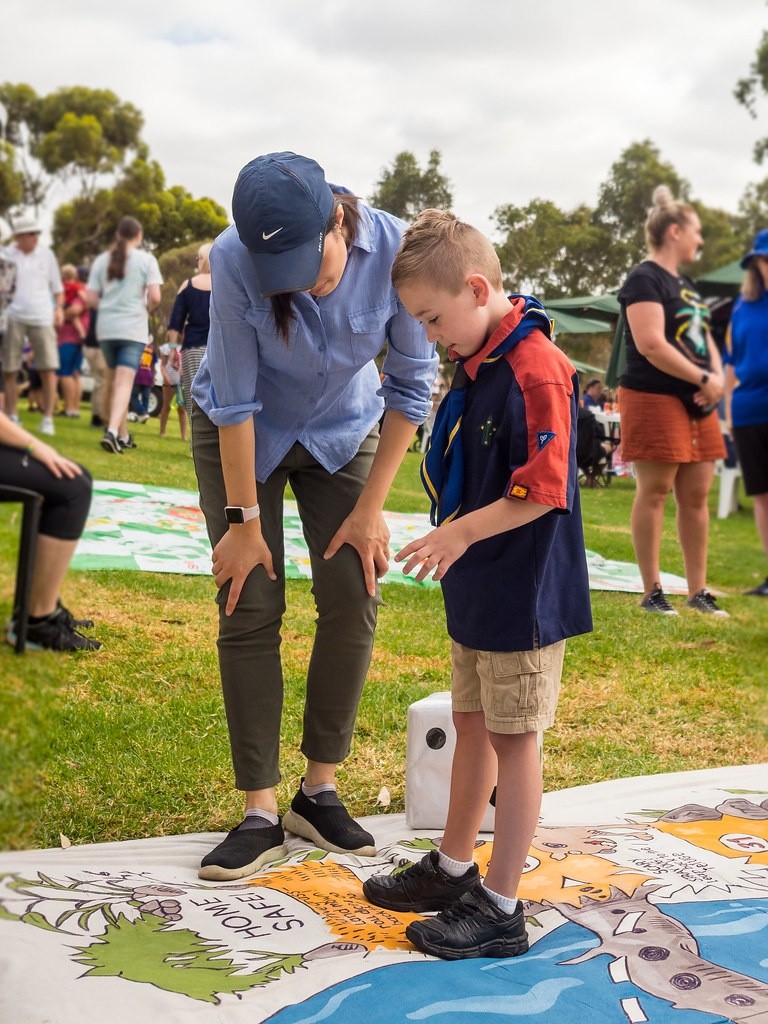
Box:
[701,371,708,384]
[224,505,259,524]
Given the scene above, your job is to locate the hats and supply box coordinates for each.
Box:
[741,228,768,268]
[13,213,43,236]
[232,152,334,297]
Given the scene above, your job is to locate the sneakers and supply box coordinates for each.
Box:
[363,850,480,913]
[282,777,377,856]
[688,591,731,619]
[8,617,102,654]
[406,886,529,961]
[51,600,92,629]
[101,433,124,456]
[118,436,137,450]
[197,814,288,880]
[642,583,678,619]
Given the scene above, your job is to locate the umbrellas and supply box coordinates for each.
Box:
[536,258,745,336]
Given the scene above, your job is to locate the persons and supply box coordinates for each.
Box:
[168,243,213,452]
[0,403,103,657]
[23,265,184,438]
[359,207,596,961]
[573,377,617,477]
[607,184,729,624]
[84,216,164,454]
[0,215,61,435]
[188,151,442,882]
[726,232,768,597]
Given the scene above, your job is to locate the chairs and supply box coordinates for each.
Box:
[0,486,43,654]
[578,440,609,487]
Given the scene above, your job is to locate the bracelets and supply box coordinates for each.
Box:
[28,439,38,452]
[169,344,177,348]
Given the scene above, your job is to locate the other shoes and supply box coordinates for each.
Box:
[140,414,149,425]
[745,577,768,598]
[93,415,103,426]
[128,412,138,422]
[41,417,56,436]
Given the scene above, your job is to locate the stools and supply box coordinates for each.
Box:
[718,469,744,518]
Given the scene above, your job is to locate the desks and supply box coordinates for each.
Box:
[595,408,621,485]
